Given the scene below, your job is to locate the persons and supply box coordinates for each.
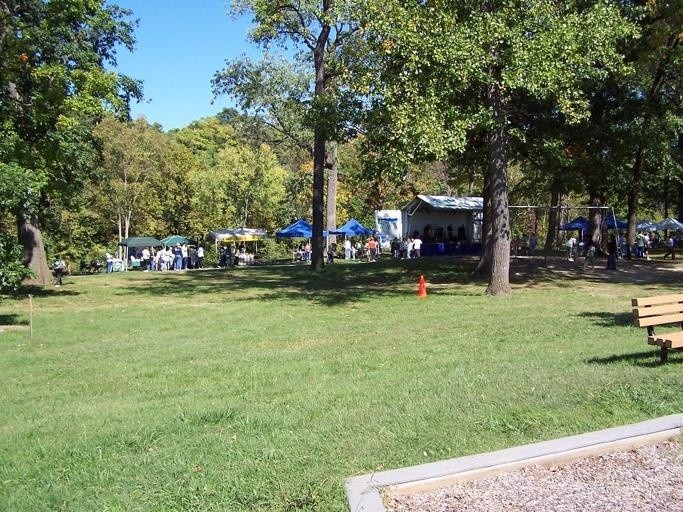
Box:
[434,226,444,242]
[293,239,312,262]
[423,222,432,240]
[325,236,423,266]
[79,240,245,273]
[566,229,683,271]
[52,254,63,288]
[509,231,536,258]
[446,224,454,241]
[457,224,466,241]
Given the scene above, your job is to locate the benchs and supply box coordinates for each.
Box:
[631,294,681,361]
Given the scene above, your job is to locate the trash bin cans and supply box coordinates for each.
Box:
[636,246,643,258]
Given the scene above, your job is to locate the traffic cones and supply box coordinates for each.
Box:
[417,274,428,298]
